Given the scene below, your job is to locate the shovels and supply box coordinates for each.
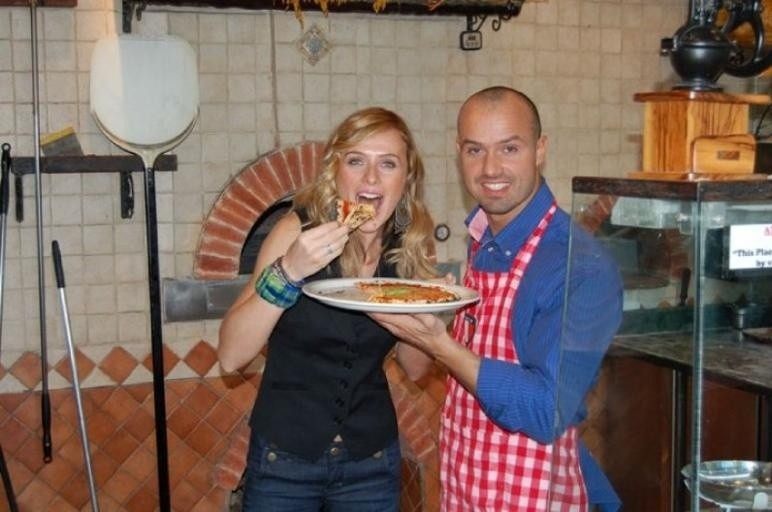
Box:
[89,32,201,512]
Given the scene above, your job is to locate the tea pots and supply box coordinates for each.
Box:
[664,13,740,94]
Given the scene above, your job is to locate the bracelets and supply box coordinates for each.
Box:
[255,256,306,309]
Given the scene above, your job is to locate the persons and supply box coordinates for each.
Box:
[216,107,458,512]
[365,85,623,512]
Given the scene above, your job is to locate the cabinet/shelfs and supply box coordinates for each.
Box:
[549,174,771,510]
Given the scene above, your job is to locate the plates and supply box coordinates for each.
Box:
[684,460,768,508]
[302,277,481,316]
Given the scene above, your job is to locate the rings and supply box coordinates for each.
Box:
[327,245,332,253]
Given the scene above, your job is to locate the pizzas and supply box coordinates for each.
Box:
[333,197,375,233]
[356,280,460,305]
